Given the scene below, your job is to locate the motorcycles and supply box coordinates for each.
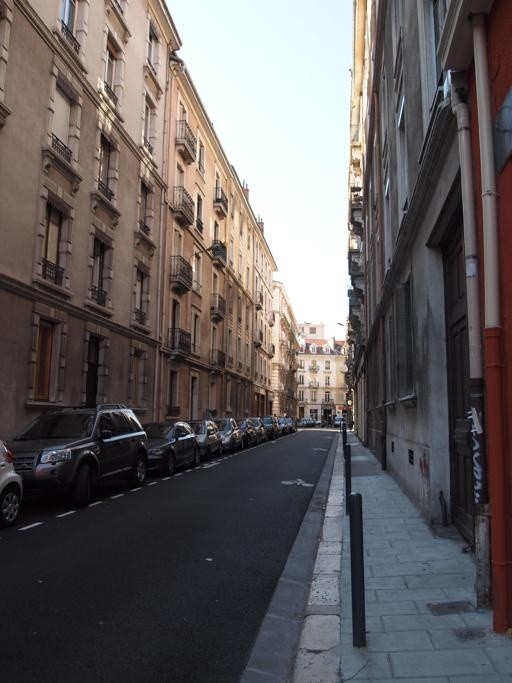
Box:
[322,419,332,428]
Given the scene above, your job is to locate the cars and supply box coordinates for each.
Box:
[334,415,345,428]
[138,421,202,478]
[1,437,25,528]
[187,415,321,462]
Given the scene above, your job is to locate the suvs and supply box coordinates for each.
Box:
[10,401,151,511]
